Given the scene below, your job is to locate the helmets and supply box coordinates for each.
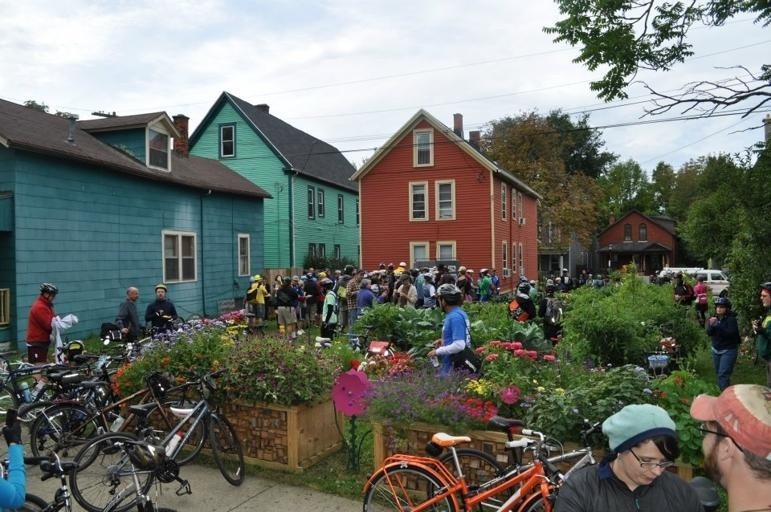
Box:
[423,272,433,280]
[714,297,732,308]
[436,283,463,298]
[318,277,334,286]
[38,282,59,295]
[478,267,489,275]
[518,281,531,291]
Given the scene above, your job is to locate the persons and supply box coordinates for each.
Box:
[116,286,139,342]
[553,404,703,511]
[649,269,672,286]
[294,253,316,331]
[511,283,537,323]
[275,277,298,341]
[144,283,178,333]
[274,275,283,298]
[380,261,500,307]
[543,267,609,292]
[690,383,771,512]
[334,263,378,332]
[1,409,25,511]
[673,271,694,305]
[706,298,741,390]
[263,276,271,319]
[752,281,771,385]
[251,275,267,318]
[319,272,334,294]
[427,282,469,378]
[537,289,564,346]
[693,277,708,328]
[320,278,337,341]
[316,267,334,328]
[26,283,77,364]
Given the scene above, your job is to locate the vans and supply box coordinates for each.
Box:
[697,270,731,298]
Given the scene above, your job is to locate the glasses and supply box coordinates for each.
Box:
[628,447,674,470]
[697,422,744,454]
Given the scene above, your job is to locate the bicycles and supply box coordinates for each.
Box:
[426,416,603,512]
[10,451,72,512]
[359,325,396,362]
[31,372,208,474]
[17,355,125,464]
[25,460,177,511]
[362,429,560,512]
[124,341,140,363]
[0,360,99,447]
[69,367,245,511]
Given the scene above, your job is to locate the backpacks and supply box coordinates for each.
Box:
[507,300,528,323]
[694,289,707,304]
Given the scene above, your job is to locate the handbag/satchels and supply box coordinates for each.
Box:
[452,310,482,376]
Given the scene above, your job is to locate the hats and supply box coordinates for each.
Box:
[673,272,684,279]
[690,383,771,462]
[696,274,705,280]
[457,265,468,272]
[759,281,771,291]
[282,276,292,286]
[253,274,263,283]
[562,268,568,272]
[317,271,328,279]
[601,403,679,455]
[248,275,256,284]
[292,274,300,283]
[466,269,474,274]
[399,260,408,267]
[153,283,169,293]
[544,285,556,294]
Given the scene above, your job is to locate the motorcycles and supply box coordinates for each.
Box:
[57,337,94,376]
[314,336,362,360]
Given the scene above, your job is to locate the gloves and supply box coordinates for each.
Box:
[2,421,23,446]
